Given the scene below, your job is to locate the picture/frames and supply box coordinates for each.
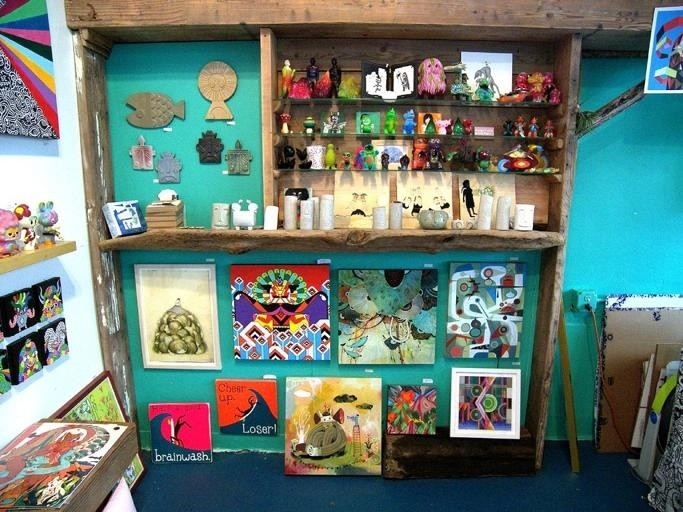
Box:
[643,4,683,95]
[42,368,147,494]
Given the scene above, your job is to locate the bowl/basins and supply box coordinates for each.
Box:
[445,119,471,134]
[418,210,447,230]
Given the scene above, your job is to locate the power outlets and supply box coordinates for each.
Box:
[576,290,597,312]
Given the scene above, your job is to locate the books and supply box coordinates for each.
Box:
[100,198,184,238]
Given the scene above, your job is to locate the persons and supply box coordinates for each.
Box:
[461,179,477,217]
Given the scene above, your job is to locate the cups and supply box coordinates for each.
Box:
[210,202,232,230]
[476,194,512,231]
[305,144,325,170]
[451,220,463,229]
[261,195,403,230]
[465,217,474,230]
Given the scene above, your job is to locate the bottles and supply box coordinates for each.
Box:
[452,117,463,136]
